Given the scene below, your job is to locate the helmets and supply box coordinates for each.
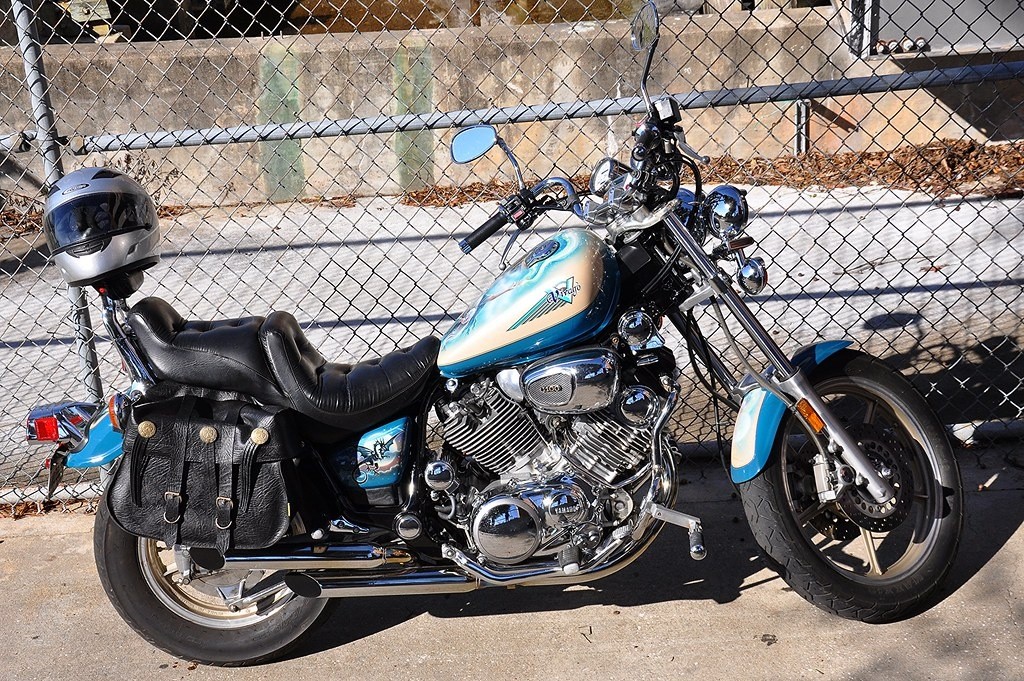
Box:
[43,168,160,286]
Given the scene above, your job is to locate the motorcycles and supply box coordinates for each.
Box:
[23,1,966,669]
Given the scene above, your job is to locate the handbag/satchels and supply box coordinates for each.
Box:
[104,389,316,556]
